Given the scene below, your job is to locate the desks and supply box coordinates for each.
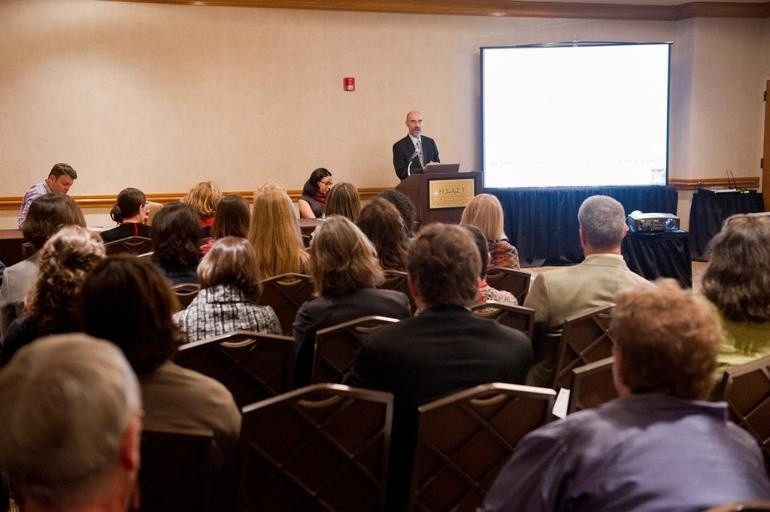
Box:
[484,186,678,264]
[689,187,765,263]
[621,229,692,289]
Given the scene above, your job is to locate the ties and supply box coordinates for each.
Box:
[415,138,425,166]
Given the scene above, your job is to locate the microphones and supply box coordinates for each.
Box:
[409,148,420,162]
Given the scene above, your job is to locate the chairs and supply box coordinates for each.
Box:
[141,430,236,511]
[564,354,621,416]
[253,272,318,332]
[310,315,402,403]
[375,271,417,315]
[473,305,535,341]
[487,267,531,306]
[170,283,203,307]
[175,332,296,414]
[234,382,393,511]
[410,382,556,512]
[104,235,155,257]
[722,355,770,465]
[549,304,624,418]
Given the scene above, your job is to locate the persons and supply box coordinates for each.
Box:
[478,278,769,512]
[525,194,661,387]
[697,212,770,359]
[1,164,534,512]
[393,112,439,180]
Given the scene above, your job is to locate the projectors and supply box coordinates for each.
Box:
[626,212,681,233]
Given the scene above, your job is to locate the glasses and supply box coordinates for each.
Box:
[321,181,333,187]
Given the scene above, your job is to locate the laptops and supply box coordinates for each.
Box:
[426,163,460,173]
